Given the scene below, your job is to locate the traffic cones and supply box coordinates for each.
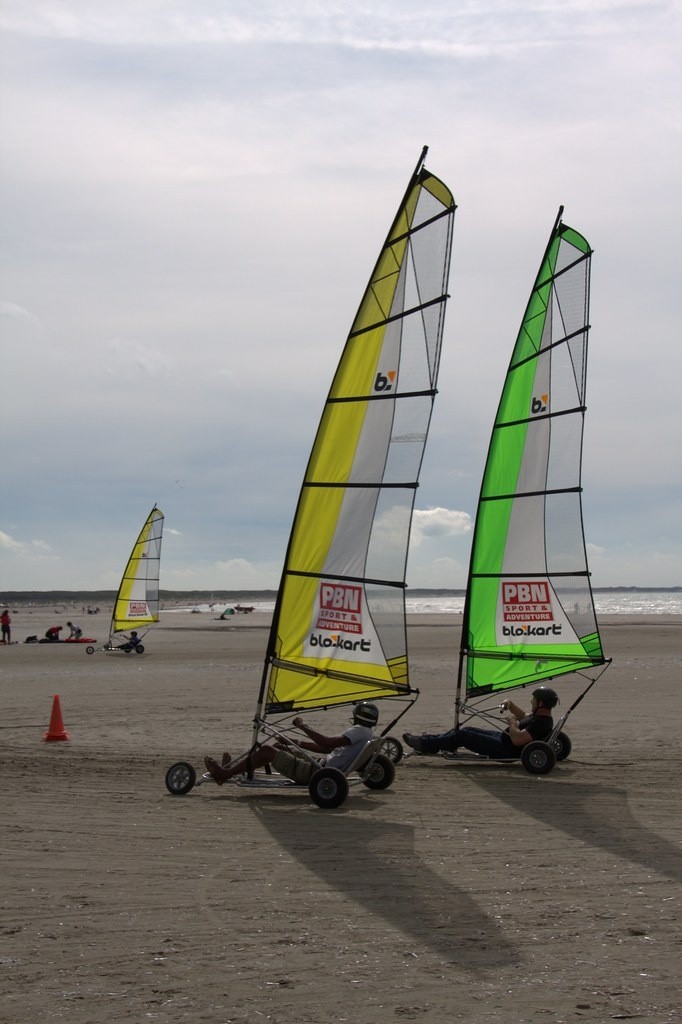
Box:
[45,694,68,742]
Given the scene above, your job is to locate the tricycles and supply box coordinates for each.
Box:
[163,142,456,803]
[84,503,165,654]
[404,205,615,772]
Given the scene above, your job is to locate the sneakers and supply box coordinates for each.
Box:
[402,731,440,754]
[221,751,231,770]
[204,755,226,786]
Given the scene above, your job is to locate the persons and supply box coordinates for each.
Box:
[45,626,63,640]
[1,610,12,645]
[103,631,139,650]
[204,702,379,784]
[402,687,558,764]
[66,621,83,639]
[220,613,226,619]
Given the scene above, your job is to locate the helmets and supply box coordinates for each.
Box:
[351,700,379,726]
[531,685,559,709]
[130,631,137,636]
